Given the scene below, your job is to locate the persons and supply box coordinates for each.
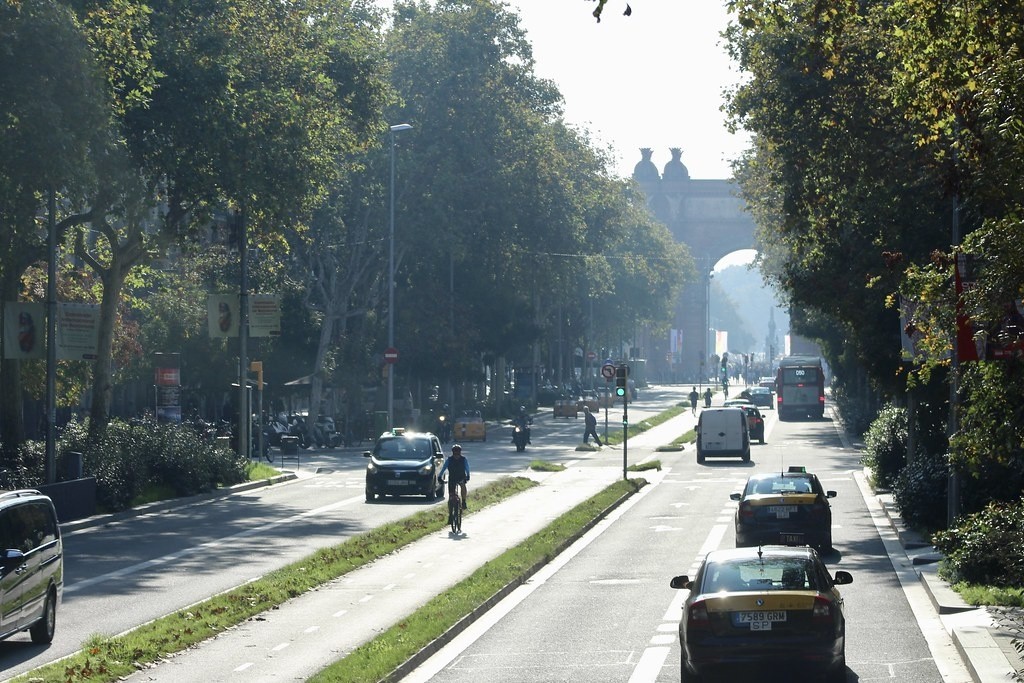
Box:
[710,357,780,385]
[438,444,469,525]
[690,387,698,412]
[511,406,532,444]
[583,406,602,446]
[703,388,712,407]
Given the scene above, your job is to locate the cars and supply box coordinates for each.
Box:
[759,377,776,391]
[0,489,65,646]
[363,428,446,501]
[749,386,774,410]
[730,466,838,553]
[724,398,766,444]
[670,543,854,683]
[553,382,637,420]
[452,409,487,442]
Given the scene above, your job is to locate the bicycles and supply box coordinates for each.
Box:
[440,477,466,533]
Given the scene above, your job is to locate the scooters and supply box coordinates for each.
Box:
[210,406,363,463]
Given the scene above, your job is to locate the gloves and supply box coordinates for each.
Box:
[438,477,443,484]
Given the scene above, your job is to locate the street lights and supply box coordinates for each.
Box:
[385,124,416,433]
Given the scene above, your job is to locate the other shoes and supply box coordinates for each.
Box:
[448,515,453,524]
[462,502,467,509]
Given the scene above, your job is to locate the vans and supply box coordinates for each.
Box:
[695,406,753,461]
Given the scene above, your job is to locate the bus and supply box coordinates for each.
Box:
[775,355,826,421]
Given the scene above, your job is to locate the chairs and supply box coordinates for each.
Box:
[782,567,806,589]
[795,484,810,493]
[714,565,746,591]
[756,481,773,494]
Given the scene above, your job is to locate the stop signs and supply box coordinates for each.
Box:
[385,347,400,365]
[586,352,597,361]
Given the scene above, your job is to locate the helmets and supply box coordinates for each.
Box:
[452,444,462,451]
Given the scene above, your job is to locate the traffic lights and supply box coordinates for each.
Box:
[721,358,727,372]
[745,354,749,363]
[615,367,628,398]
[722,378,727,393]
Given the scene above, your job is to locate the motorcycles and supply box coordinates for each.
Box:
[510,419,531,452]
